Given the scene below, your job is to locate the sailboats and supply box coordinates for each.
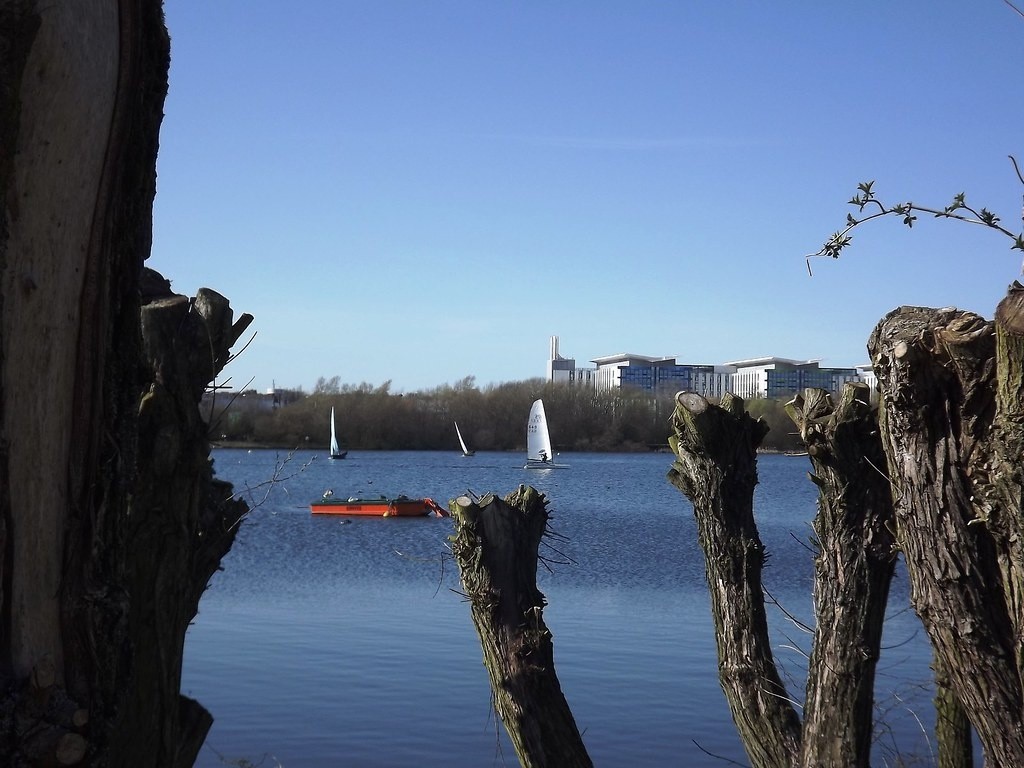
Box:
[523,398,570,470]
[328,406,347,458]
[454,421,475,458]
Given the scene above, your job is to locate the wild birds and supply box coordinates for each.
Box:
[321,490,333,500]
[348,496,361,503]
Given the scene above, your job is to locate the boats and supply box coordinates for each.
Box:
[309,491,448,517]
[782,451,810,457]
